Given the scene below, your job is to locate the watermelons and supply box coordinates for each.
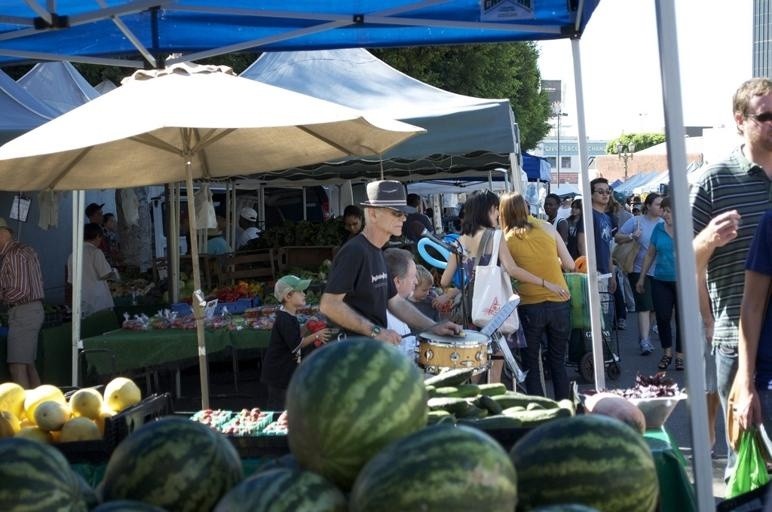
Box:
[0,338,659,512]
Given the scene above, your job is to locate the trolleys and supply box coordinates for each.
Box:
[564,271,621,382]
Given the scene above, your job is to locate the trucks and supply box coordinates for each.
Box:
[1,186,232,305]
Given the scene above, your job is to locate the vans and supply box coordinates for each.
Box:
[219,185,331,233]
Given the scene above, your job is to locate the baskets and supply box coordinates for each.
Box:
[52,385,174,464]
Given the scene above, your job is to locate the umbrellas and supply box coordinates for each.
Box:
[0,51,429,410]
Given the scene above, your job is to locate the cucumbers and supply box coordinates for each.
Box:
[420,367,569,428]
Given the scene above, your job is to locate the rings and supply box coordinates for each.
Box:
[561,289,565,293]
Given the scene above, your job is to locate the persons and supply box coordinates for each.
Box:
[402,194,433,257]
[0,229,50,388]
[693,76,771,486]
[344,205,365,245]
[237,207,262,249]
[260,272,333,409]
[734,208,771,459]
[433,187,571,383]
[67,203,118,330]
[544,177,685,372]
[207,227,230,254]
[498,192,576,399]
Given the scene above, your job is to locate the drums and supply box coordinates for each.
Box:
[418,327,492,371]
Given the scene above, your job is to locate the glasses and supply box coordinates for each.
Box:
[744,112,771,123]
[595,190,610,194]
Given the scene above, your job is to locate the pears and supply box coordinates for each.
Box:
[0,376,141,446]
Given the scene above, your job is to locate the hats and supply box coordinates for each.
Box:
[360,180,417,214]
[240,207,258,222]
[86,204,104,216]
[274,276,311,302]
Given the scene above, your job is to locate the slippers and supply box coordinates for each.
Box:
[657,355,684,369]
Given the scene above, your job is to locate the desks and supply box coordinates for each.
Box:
[79,313,336,398]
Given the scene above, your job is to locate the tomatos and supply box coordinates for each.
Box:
[196,406,288,436]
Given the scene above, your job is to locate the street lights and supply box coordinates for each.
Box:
[613,141,637,182]
[552,108,569,188]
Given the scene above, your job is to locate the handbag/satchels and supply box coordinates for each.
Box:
[462,262,519,335]
[612,239,640,273]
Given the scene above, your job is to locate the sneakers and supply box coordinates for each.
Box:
[640,338,653,354]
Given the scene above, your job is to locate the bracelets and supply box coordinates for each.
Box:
[540,276,546,288]
